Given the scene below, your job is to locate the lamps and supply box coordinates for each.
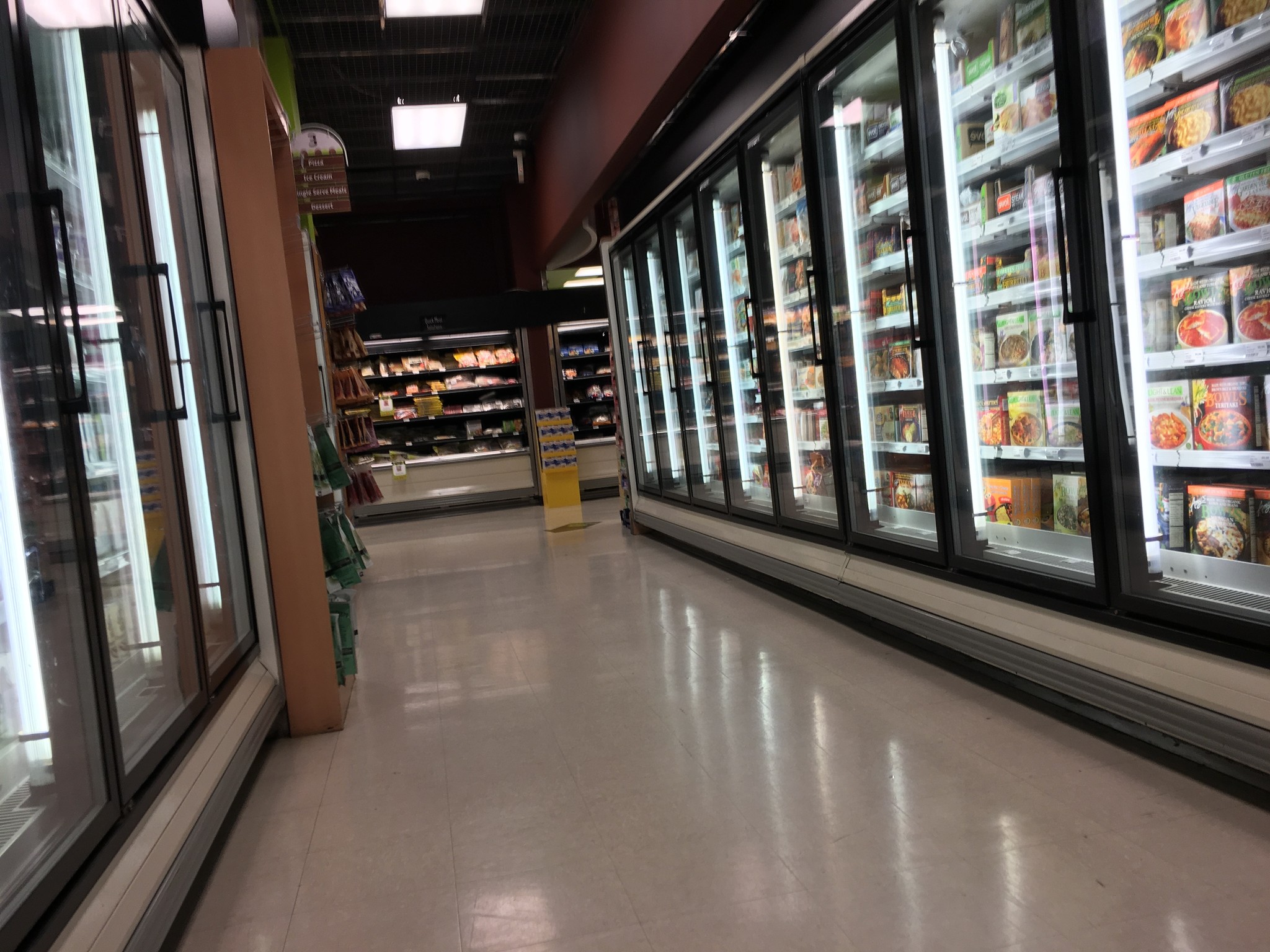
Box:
[378,0,489,152]
